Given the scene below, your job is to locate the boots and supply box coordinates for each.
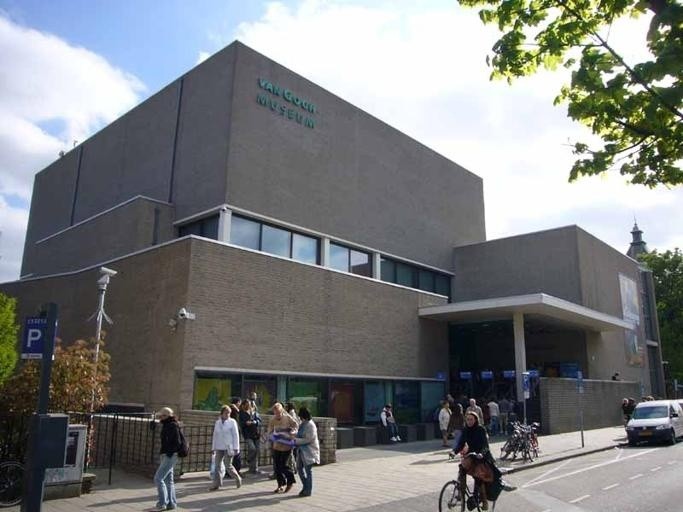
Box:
[455,479,466,500]
[479,482,488,509]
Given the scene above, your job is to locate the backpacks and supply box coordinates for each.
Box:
[169,422,189,457]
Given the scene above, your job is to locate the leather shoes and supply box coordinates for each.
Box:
[502,481,518,491]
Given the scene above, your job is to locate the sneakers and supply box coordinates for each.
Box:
[390,435,402,442]
[208,468,310,496]
[148,501,175,511]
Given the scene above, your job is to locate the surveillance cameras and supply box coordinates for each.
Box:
[99,266,118,277]
[180,307,186,317]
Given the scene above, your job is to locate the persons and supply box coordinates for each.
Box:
[448,410,490,511]
[225,391,321,496]
[432,392,517,447]
[622,395,654,427]
[612,372,620,381]
[152,406,181,512]
[380,403,402,442]
[208,405,242,490]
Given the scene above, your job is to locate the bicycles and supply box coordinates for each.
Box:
[439,449,498,511]
[499,419,541,462]
[0,457,27,508]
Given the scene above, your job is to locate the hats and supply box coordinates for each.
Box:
[155,406,173,417]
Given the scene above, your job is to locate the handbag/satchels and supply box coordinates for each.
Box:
[210,451,227,480]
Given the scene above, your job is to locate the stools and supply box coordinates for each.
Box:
[378,425,396,444]
[337,427,354,447]
[355,425,378,446]
[417,423,435,440]
[399,424,417,441]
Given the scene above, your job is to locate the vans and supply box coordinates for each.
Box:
[624,398,683,447]
